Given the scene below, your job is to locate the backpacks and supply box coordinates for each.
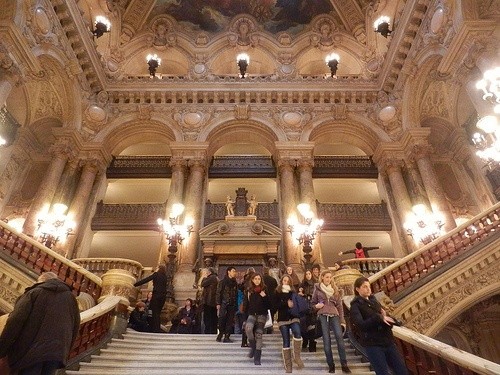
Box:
[356,246,365,259]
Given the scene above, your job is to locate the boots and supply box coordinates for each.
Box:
[292,336,304,367]
[282,346,292,373]
[254,349,261,365]
[248,339,256,358]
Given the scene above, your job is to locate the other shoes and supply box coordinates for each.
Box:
[224,333,230,342]
[216,332,223,342]
[329,363,335,373]
[342,367,351,373]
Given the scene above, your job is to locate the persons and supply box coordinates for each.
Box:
[273,274,305,373]
[282,267,300,291]
[0,271,80,375]
[171,298,196,334]
[129,264,168,334]
[312,270,351,374]
[216,267,238,343]
[239,265,278,347]
[225,196,235,215]
[350,276,407,375]
[302,270,317,352]
[311,267,320,282]
[338,242,382,273]
[245,273,271,365]
[334,261,348,271]
[200,267,220,334]
[247,196,258,215]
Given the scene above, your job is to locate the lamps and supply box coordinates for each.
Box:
[286,201,325,253]
[146,53,162,77]
[324,52,341,78]
[34,201,78,249]
[92,15,112,38]
[157,202,194,262]
[237,52,249,79]
[373,16,392,38]
[403,202,446,245]
[470,67,500,164]
